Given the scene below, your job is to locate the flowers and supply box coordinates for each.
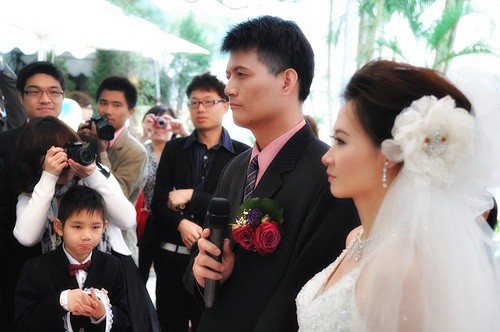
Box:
[380,94,477,186]
[231,197,284,256]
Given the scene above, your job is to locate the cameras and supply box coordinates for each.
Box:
[59,140,96,169]
[149,116,168,128]
[81,115,116,141]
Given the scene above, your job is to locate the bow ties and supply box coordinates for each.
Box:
[66,260,93,277]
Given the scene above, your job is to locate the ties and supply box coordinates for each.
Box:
[244,156,260,204]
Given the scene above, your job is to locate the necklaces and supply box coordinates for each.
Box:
[347,228,368,260]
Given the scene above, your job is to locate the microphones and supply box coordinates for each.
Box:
[203,196,228,310]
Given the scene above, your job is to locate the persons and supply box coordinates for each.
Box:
[151,74,250,332]
[190,15,362,332]
[77,75,149,205]
[134,107,190,288]
[12,116,159,332]
[296,59,500,332]
[13,186,131,332]
[0,62,65,332]
[69,91,93,124]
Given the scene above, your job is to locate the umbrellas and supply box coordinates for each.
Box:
[0,0,210,62]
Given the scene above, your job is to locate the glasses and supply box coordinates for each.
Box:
[186,99,224,109]
[24,87,64,99]
